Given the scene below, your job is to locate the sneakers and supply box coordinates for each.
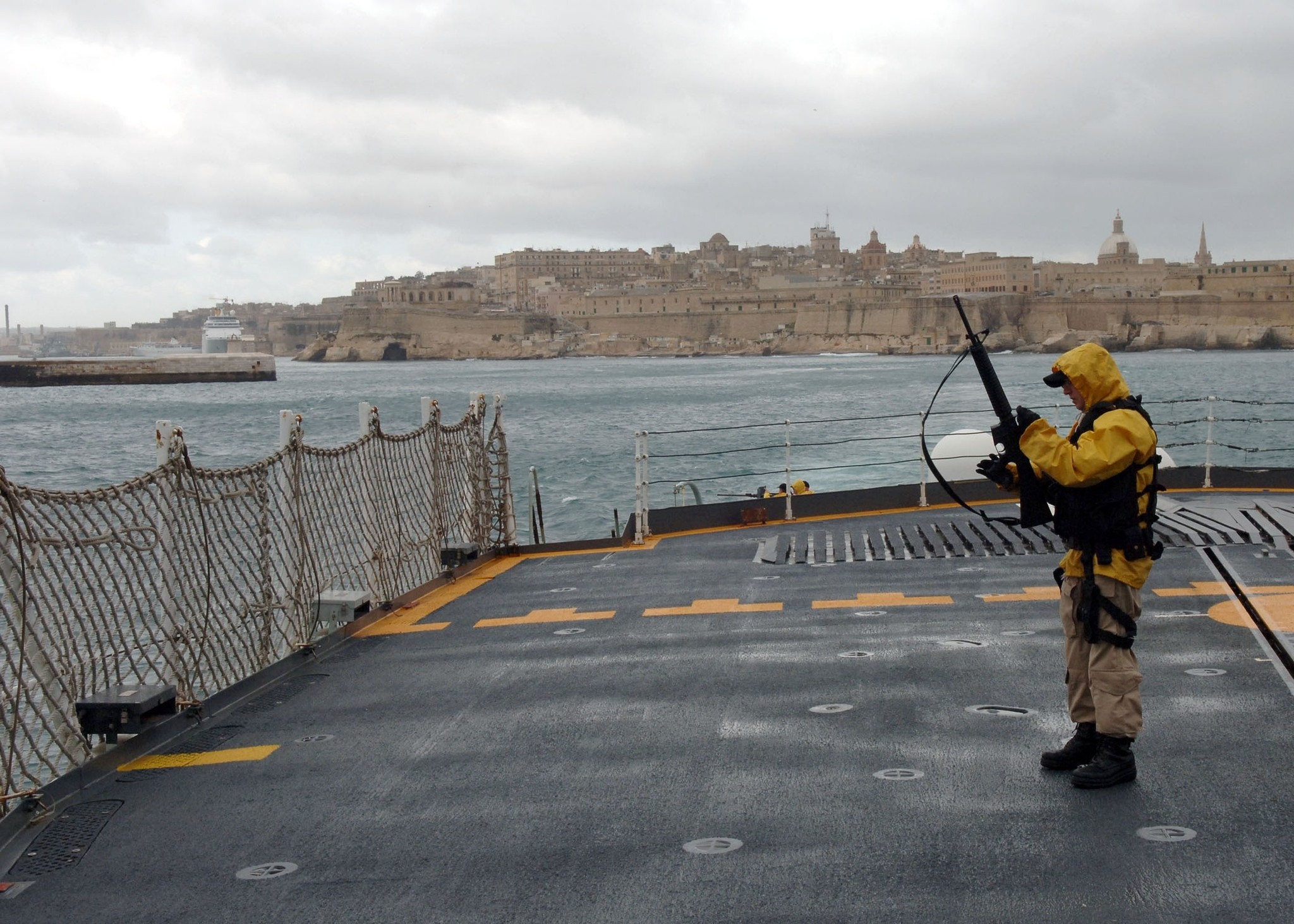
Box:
[1040,722,1104,771]
[1071,736,1137,788]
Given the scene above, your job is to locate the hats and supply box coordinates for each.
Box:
[777,483,786,489]
[1043,370,1066,388]
[802,481,809,488]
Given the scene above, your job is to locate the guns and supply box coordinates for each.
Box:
[953,295,1054,529]
[717,493,779,498]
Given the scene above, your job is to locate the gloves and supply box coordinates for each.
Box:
[975,453,1014,489]
[1016,405,1041,432]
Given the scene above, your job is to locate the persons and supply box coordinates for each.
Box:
[975,342,1158,790]
[763,480,816,498]
